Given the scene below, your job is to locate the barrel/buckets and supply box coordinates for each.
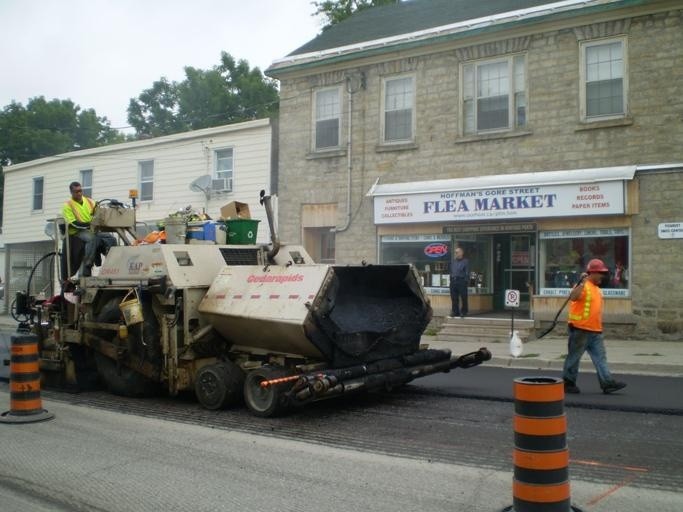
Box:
[165,220,186,244]
[119,289,144,325]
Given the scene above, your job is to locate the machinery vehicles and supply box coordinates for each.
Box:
[9,188,491,417]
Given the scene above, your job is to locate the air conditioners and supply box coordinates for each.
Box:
[211,177,232,192]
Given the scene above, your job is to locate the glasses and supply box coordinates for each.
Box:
[72,190,82,193]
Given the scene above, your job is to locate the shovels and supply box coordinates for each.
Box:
[538,274,586,339]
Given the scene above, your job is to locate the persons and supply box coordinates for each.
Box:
[449,246,474,319]
[562,259,629,393]
[59,179,117,276]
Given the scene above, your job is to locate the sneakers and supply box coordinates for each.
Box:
[83,263,91,277]
[603,381,627,393]
[564,382,580,393]
[449,310,467,317]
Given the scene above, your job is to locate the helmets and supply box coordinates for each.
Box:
[586,259,609,273]
